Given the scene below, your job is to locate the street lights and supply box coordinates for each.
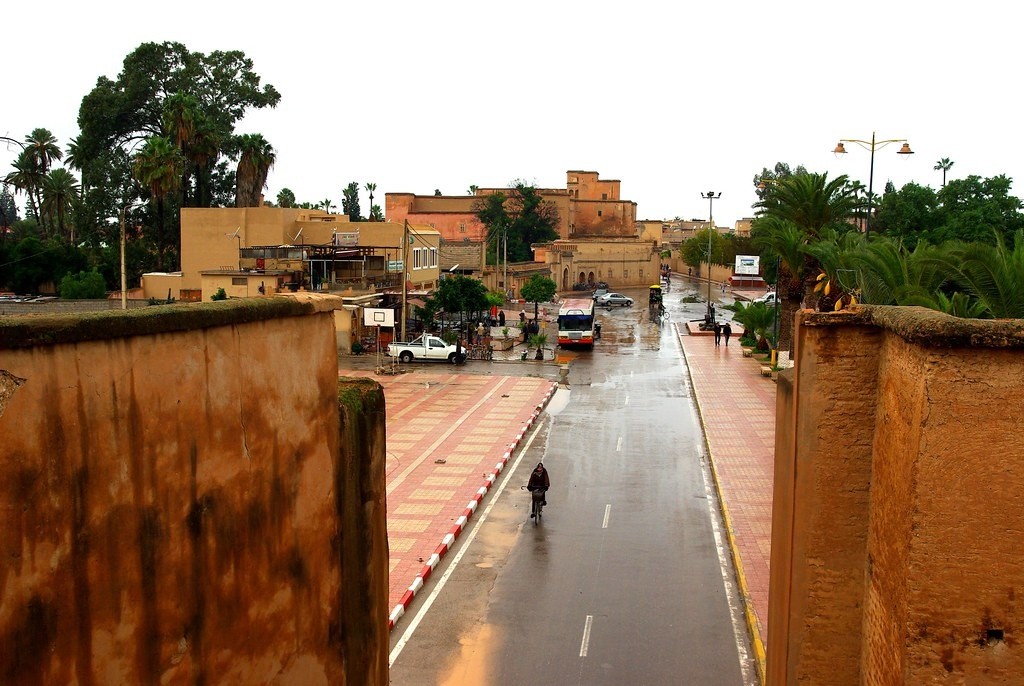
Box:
[120,197,151,311]
[699,190,723,323]
[830,130,915,242]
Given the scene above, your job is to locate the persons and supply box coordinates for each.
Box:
[688,267,700,281]
[506,289,514,302]
[767,285,772,293]
[660,262,672,287]
[549,291,559,305]
[710,302,715,323]
[723,323,731,346]
[454,339,466,366]
[468,310,506,347]
[650,288,666,316]
[713,323,721,346]
[527,462,550,518]
[519,309,539,343]
[351,339,363,357]
[720,277,732,293]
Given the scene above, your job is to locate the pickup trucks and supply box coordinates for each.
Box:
[387,330,468,365]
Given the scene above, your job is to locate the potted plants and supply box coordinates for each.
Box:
[528,332,548,361]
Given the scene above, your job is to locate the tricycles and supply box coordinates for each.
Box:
[648,285,663,305]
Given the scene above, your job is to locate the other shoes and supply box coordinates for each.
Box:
[543,502,546,506]
[531,513,534,518]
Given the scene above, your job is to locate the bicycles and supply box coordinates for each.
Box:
[527,485,546,527]
[655,308,671,320]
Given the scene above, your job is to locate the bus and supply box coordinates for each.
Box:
[556,298,598,348]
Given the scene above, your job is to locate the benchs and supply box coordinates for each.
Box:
[743,349,752,357]
[761,367,772,377]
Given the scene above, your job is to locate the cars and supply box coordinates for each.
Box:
[592,289,609,303]
[596,293,634,308]
[754,291,782,306]
